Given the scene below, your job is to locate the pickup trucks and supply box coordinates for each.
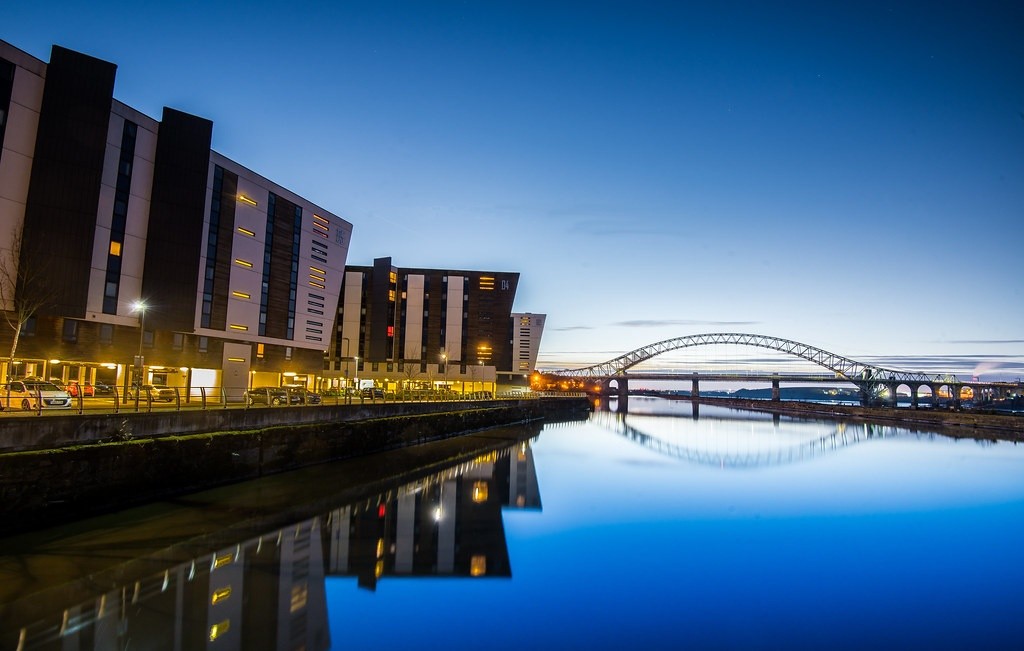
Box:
[326,389,345,396]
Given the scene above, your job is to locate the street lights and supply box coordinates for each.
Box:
[354,357,359,391]
[343,337,349,404]
[442,355,451,400]
[479,361,484,399]
[133,301,146,412]
[524,374,528,397]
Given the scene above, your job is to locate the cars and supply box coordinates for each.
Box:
[67,379,94,398]
[127,384,176,402]
[360,388,385,400]
[346,388,357,396]
[94,382,112,395]
[132,382,142,389]
[24,376,68,395]
[243,387,301,406]
[462,390,492,399]
[279,387,321,404]
[0,379,73,412]
